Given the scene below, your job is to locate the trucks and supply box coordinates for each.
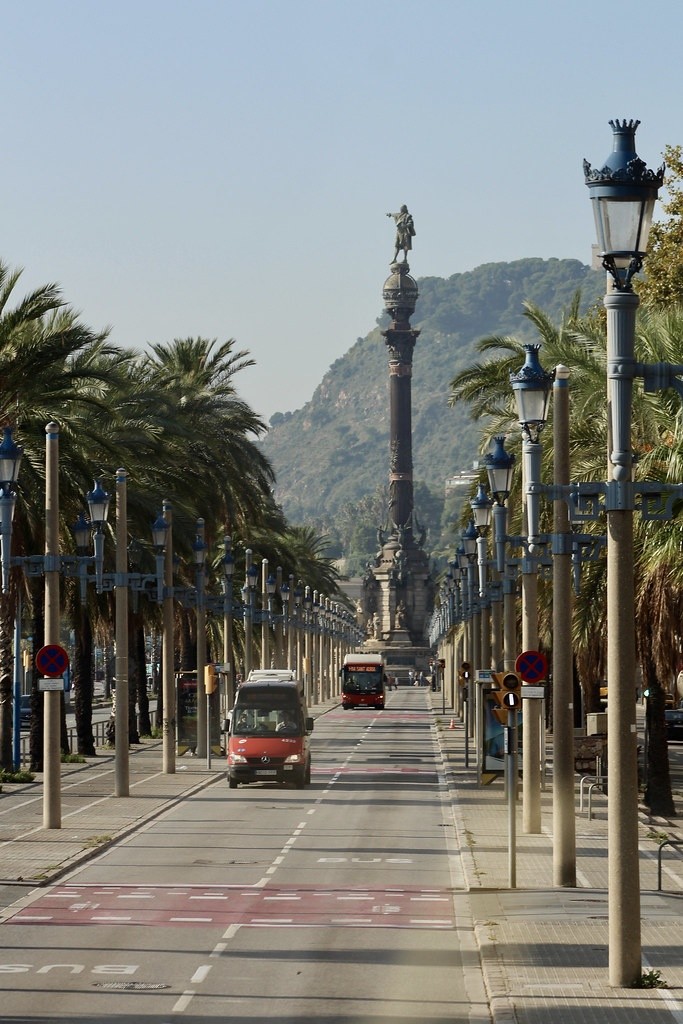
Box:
[220,669,315,789]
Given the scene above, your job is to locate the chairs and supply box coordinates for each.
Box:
[22,701,27,706]
[277,711,292,725]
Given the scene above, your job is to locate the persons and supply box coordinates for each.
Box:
[387,674,392,691]
[409,670,414,685]
[386,204,416,265]
[397,600,406,619]
[276,712,297,732]
[238,714,247,729]
[346,678,354,685]
[394,676,398,689]
[367,612,380,639]
[376,523,426,550]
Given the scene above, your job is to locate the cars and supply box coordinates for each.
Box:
[666,709,683,744]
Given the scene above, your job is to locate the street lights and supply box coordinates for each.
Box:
[59,467,163,797]
[147,498,367,775]
[0,420,65,830]
[509,119,665,989]
[427,364,607,889]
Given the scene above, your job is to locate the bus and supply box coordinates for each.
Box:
[338,654,387,710]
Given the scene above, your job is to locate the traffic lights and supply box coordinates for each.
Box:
[437,658,446,669]
[490,671,523,710]
[457,661,472,680]
[490,707,508,726]
